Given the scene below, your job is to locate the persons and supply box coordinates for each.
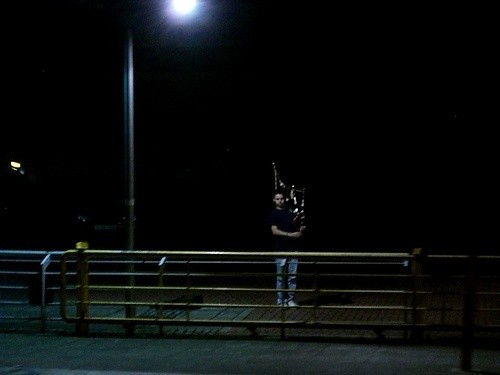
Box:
[270,189,303,308]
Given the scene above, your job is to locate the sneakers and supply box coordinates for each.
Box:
[278,300,299,309]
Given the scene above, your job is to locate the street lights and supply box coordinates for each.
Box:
[124,0,204,327]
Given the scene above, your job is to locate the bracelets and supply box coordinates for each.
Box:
[285,232,287,236]
